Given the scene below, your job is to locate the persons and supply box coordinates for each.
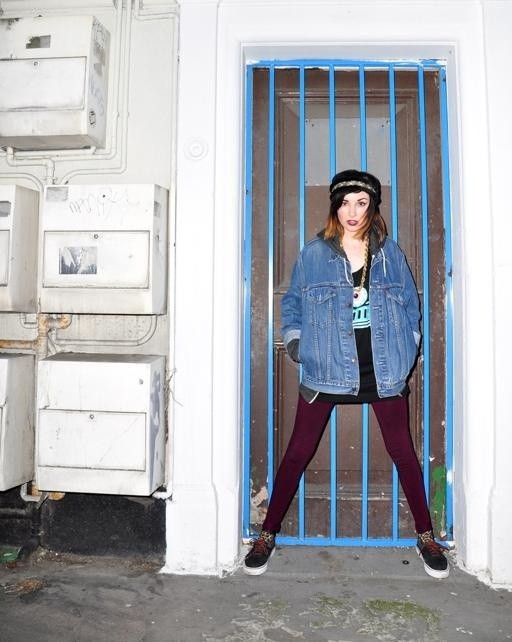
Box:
[242,169,454,581]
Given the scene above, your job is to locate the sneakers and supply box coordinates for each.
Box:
[242,537,276,576]
[416,536,450,578]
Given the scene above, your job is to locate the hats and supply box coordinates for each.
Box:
[330,170,381,205]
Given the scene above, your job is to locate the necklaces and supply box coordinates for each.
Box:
[337,232,370,292]
